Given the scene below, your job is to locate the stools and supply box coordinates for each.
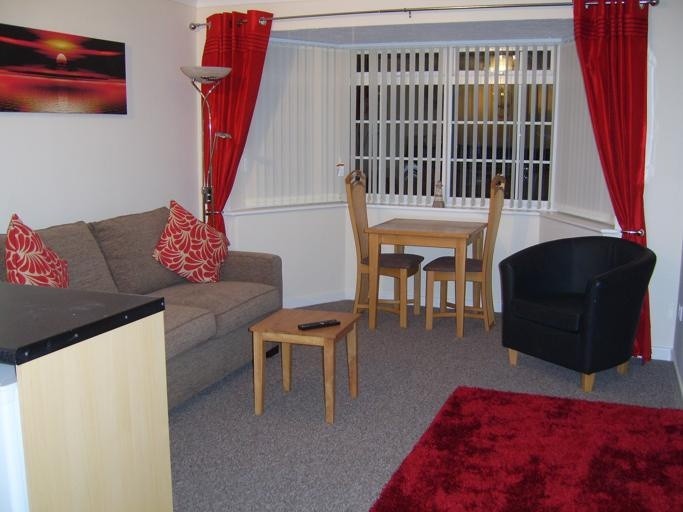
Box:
[249,306,360,425]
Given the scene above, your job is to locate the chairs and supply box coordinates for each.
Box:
[423,174,507,333]
[345,169,423,328]
[496,234,656,396]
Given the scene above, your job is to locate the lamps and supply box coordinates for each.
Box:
[179,62,233,233]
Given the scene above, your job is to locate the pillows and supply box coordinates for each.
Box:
[3,215,72,293]
[156,199,231,286]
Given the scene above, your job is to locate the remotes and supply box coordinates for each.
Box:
[297,318,341,331]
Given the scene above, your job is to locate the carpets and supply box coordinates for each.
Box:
[368,382,682,512]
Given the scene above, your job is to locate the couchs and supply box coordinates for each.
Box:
[0,204,283,418]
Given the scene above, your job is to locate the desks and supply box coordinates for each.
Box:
[362,218,488,339]
